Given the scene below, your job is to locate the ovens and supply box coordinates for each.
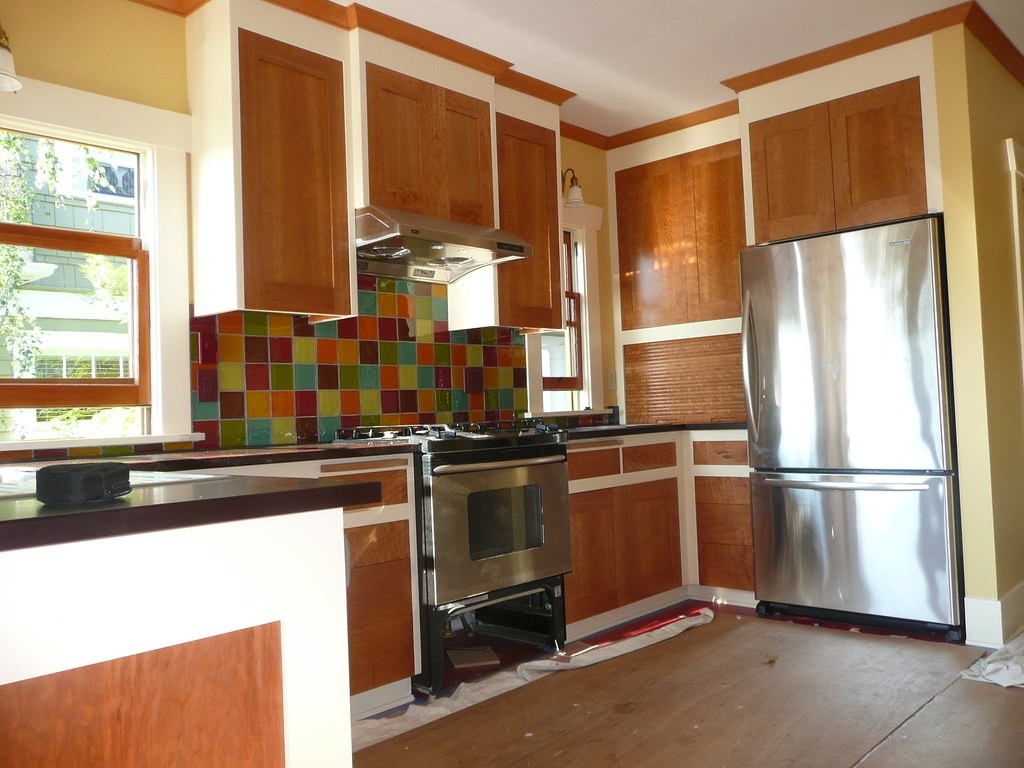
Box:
[412,443,573,694]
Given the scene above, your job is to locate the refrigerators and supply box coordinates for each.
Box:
[738,218,965,644]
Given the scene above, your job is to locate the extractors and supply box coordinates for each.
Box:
[355,206,532,284]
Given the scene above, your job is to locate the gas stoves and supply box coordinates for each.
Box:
[331,419,568,452]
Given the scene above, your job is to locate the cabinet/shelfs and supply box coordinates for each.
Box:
[614,134,753,427]
[749,76,931,248]
[184,0,566,335]
[565,429,760,645]
[0,451,423,768]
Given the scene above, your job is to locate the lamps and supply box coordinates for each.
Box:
[561,169,584,208]
[0,25,22,92]
